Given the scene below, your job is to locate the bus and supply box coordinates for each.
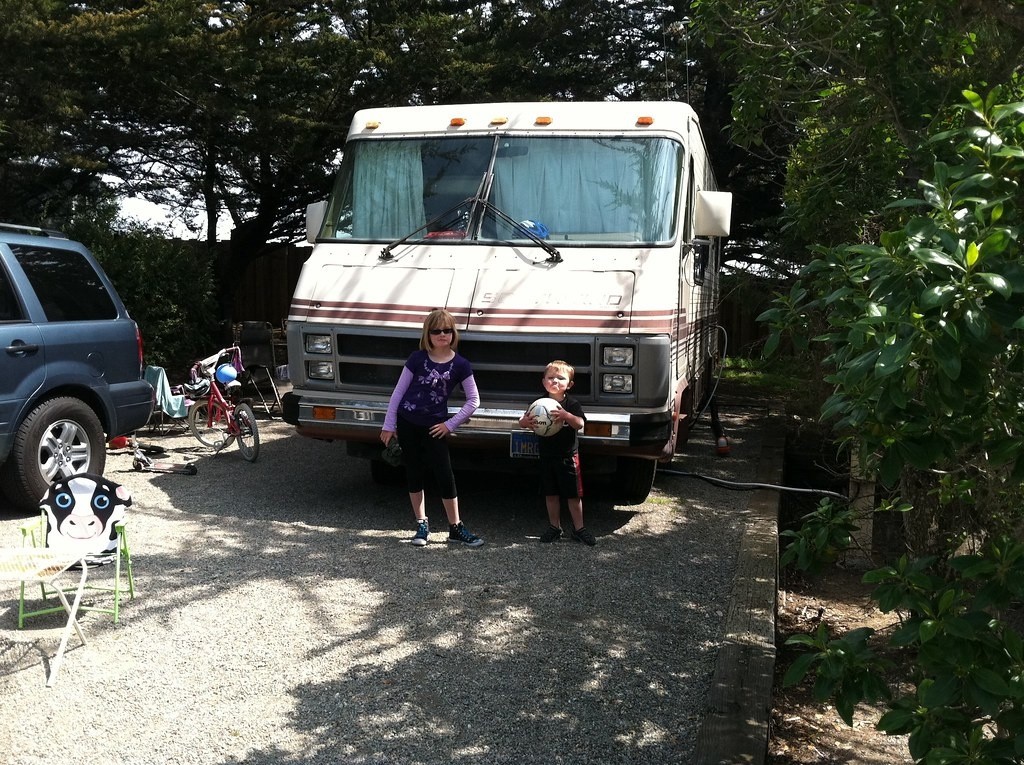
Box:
[282,100,733,471]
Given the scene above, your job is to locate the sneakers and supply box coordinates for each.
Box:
[571,524,596,547]
[412,517,430,546]
[539,525,564,543]
[447,521,484,546]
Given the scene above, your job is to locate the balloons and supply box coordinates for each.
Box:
[217,363,237,383]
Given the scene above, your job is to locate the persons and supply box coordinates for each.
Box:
[518,360,597,546]
[380,310,484,547]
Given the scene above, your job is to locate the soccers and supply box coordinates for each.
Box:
[527,398,564,437]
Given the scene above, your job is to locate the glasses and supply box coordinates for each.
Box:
[427,326,455,336]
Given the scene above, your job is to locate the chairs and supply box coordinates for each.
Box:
[18,473,135,630]
[243,364,283,420]
[144,364,196,437]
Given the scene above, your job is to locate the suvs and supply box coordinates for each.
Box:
[0,216,154,513]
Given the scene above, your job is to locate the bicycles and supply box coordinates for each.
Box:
[186,345,260,465]
